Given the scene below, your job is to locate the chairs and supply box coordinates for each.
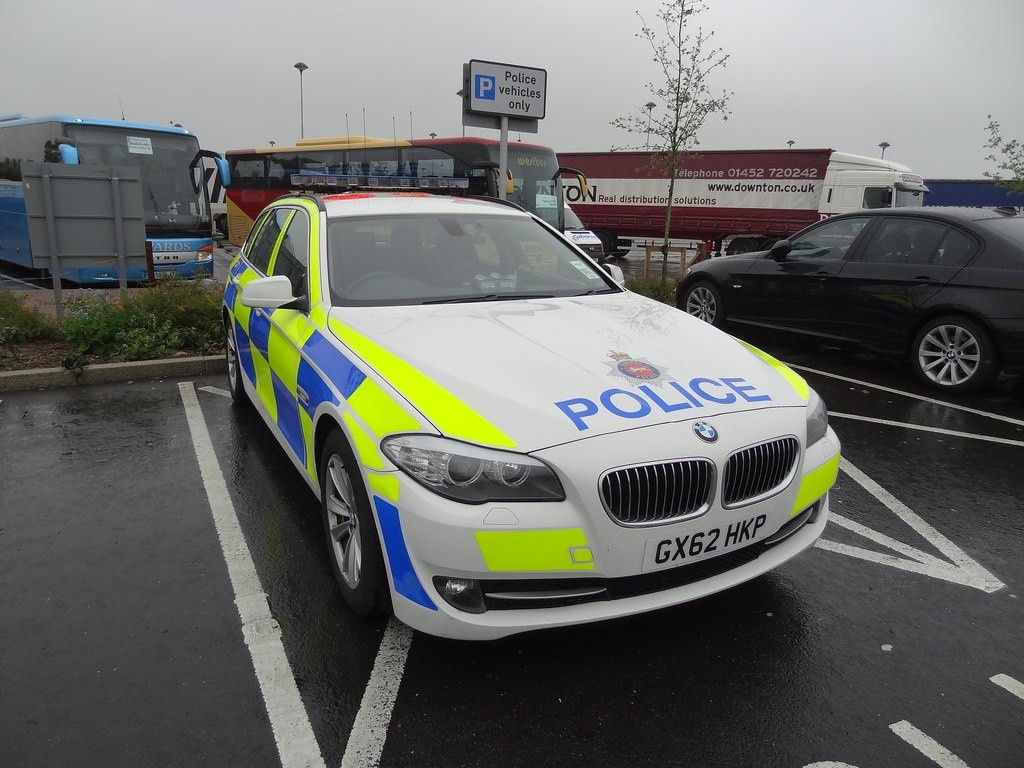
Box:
[879,232,912,262]
[386,223,449,295]
[909,231,940,263]
[331,223,379,296]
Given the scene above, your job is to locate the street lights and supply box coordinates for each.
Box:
[878,141,890,160]
[268,141,276,147]
[786,139,795,149]
[293,61,308,138]
[644,101,657,152]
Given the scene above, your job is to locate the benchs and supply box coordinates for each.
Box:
[240,175,280,187]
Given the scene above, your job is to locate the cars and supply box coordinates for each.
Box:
[219,170,843,644]
[674,204,1024,396]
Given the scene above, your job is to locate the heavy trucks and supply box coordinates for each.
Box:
[201,148,932,256]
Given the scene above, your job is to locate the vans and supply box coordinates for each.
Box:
[534,193,606,263]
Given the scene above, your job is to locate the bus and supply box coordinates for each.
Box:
[225,134,589,274]
[0,112,232,284]
